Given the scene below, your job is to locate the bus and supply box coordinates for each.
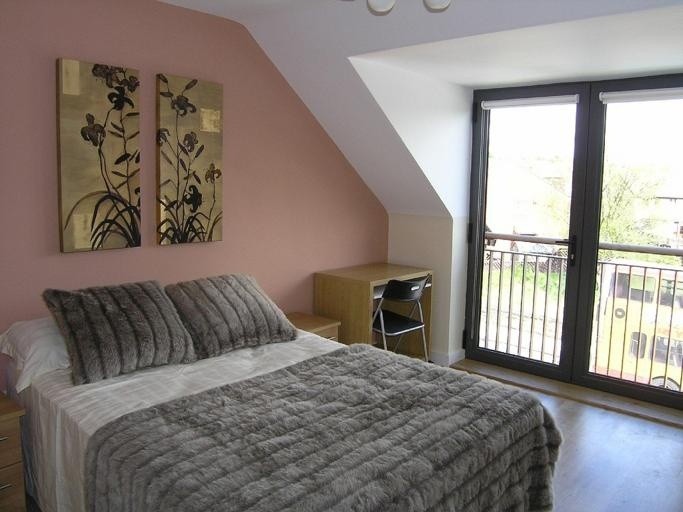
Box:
[595,259,683,393]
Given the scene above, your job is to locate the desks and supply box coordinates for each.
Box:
[312,263,434,363]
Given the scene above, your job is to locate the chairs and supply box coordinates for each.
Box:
[372,272,432,362]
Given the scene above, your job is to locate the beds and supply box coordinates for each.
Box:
[3,328,563,512]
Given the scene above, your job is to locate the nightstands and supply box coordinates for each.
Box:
[287,310,342,342]
[0,405,25,511]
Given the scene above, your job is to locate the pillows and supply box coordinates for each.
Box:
[0,315,71,395]
[164,271,296,360]
[42,280,199,385]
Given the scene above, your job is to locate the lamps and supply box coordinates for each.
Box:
[421,0,450,12]
[367,1,396,15]
